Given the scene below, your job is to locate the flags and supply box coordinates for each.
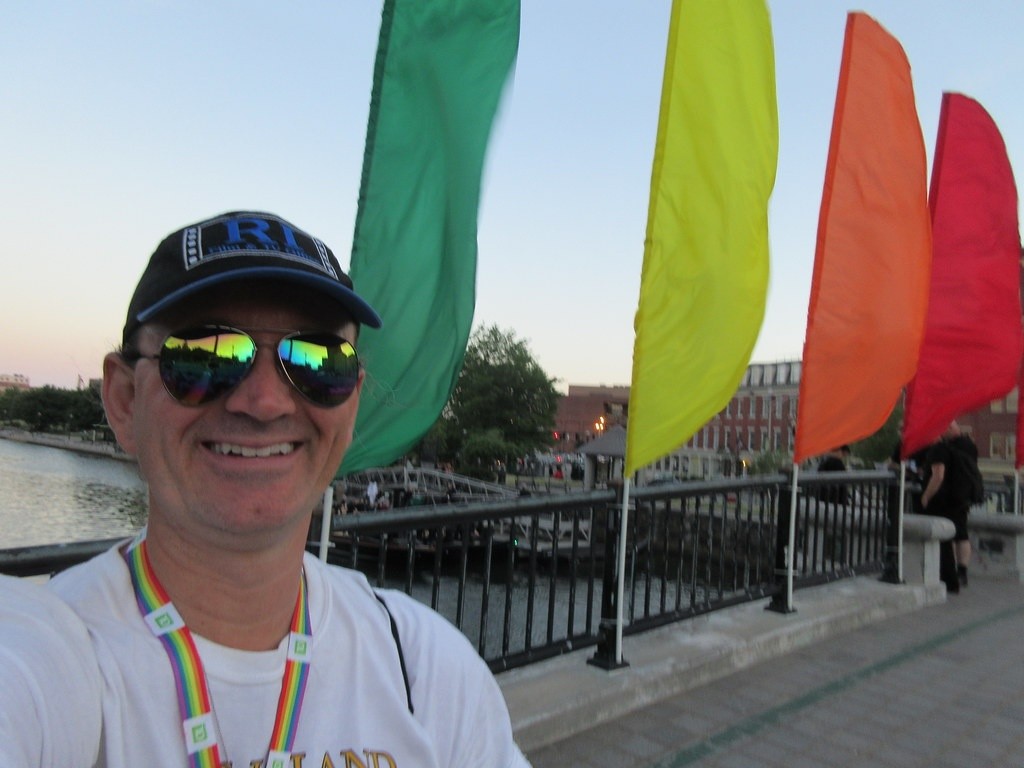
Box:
[902,93,1024,468]
[335,0,520,475]
[624,1,780,479]
[795,13,932,461]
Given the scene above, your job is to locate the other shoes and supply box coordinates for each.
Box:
[957,564,969,587]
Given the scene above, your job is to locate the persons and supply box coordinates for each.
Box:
[0,211,532,768]
[516,482,532,500]
[886,418,984,593]
[816,444,856,559]
[437,460,454,498]
[393,453,417,505]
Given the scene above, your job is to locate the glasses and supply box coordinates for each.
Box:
[136,321,362,407]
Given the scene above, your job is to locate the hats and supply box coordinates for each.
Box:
[842,445,852,456]
[120,208,384,357]
